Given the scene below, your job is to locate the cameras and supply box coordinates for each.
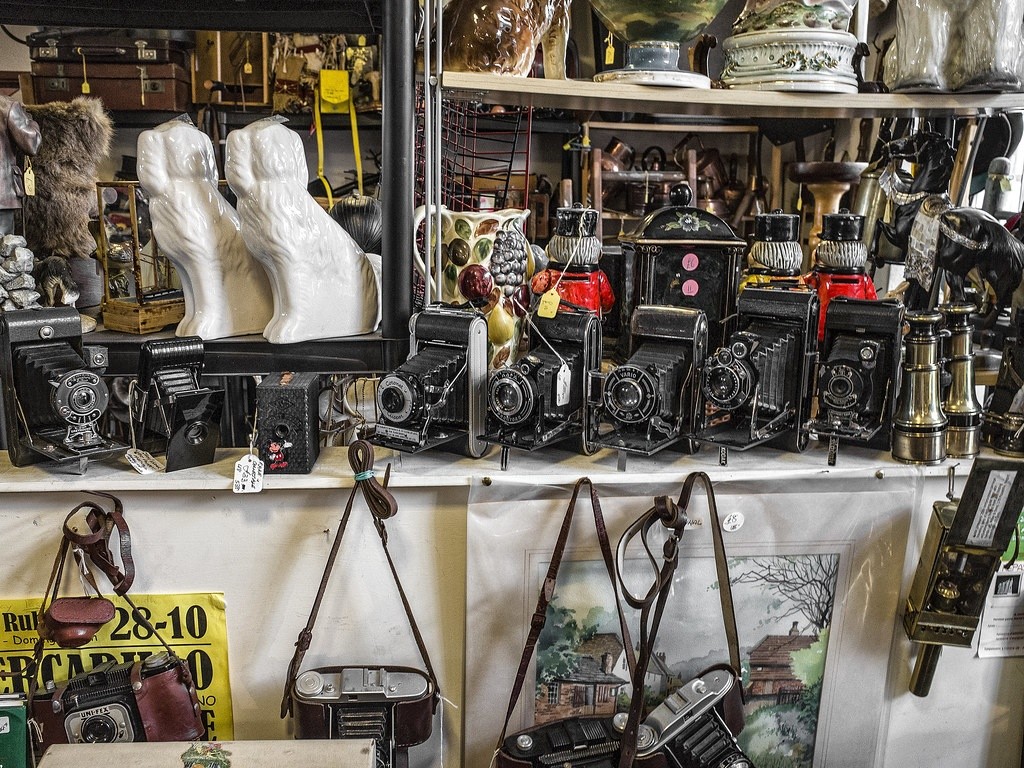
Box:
[635,669,754,768]
[295,669,432,768]
[501,712,630,768]
[31,651,205,753]
[1,289,905,480]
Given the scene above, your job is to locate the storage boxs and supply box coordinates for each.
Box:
[30,62,190,112]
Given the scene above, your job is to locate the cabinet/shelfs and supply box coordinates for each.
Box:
[0,30,1024,768]
